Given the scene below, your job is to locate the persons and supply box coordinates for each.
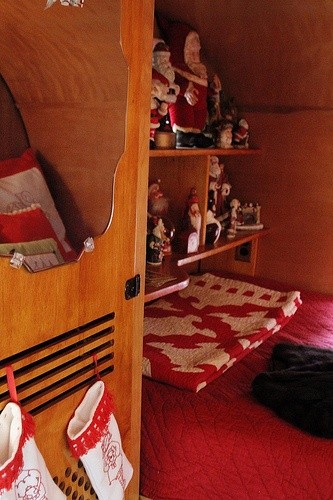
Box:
[149,29,253,151]
[145,155,267,268]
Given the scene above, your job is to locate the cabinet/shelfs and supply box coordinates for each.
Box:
[0,0,154,500]
[143,148,271,305]
